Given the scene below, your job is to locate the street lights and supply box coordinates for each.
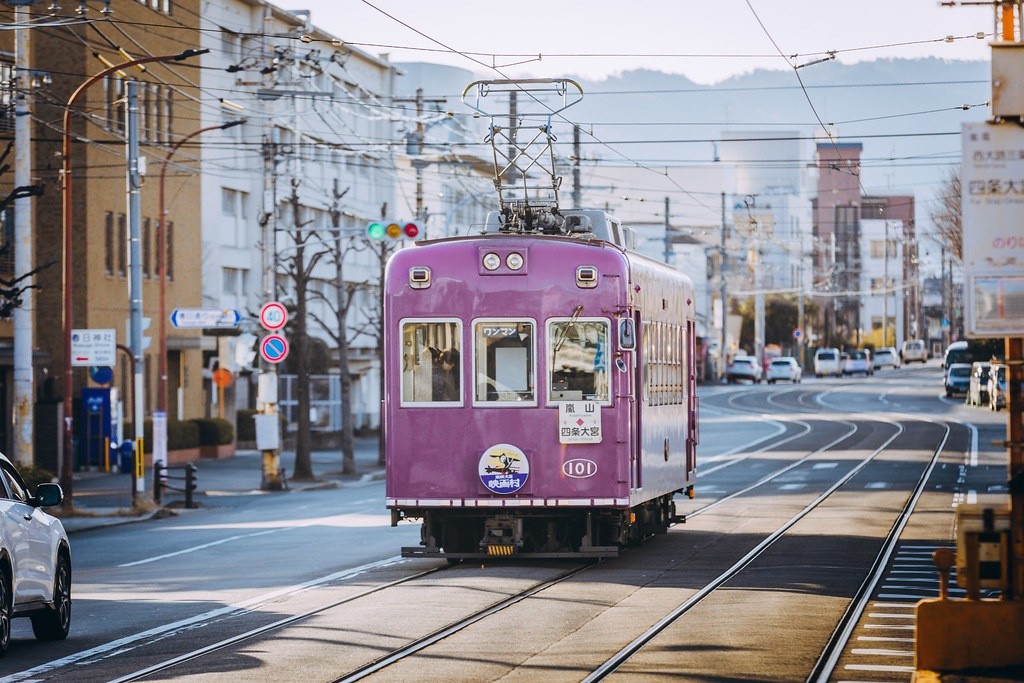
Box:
[59,48,212,516]
[151,120,248,490]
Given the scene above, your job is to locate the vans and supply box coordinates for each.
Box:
[902,340,928,365]
[940,341,968,387]
[814,348,843,379]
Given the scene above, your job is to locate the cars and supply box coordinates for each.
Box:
[944,364,972,398]
[874,346,901,370]
[982,364,1009,413]
[766,357,803,385]
[966,361,993,407]
[1,452,75,657]
[727,355,763,385]
[845,349,875,376]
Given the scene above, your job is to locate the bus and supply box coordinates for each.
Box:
[382,207,697,566]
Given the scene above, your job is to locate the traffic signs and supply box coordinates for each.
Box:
[170,308,241,328]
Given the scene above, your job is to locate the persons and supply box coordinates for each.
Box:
[440,347,460,401]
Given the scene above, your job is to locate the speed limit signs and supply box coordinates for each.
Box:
[260,303,287,331]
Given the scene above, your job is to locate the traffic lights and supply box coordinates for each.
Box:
[365,220,425,242]
